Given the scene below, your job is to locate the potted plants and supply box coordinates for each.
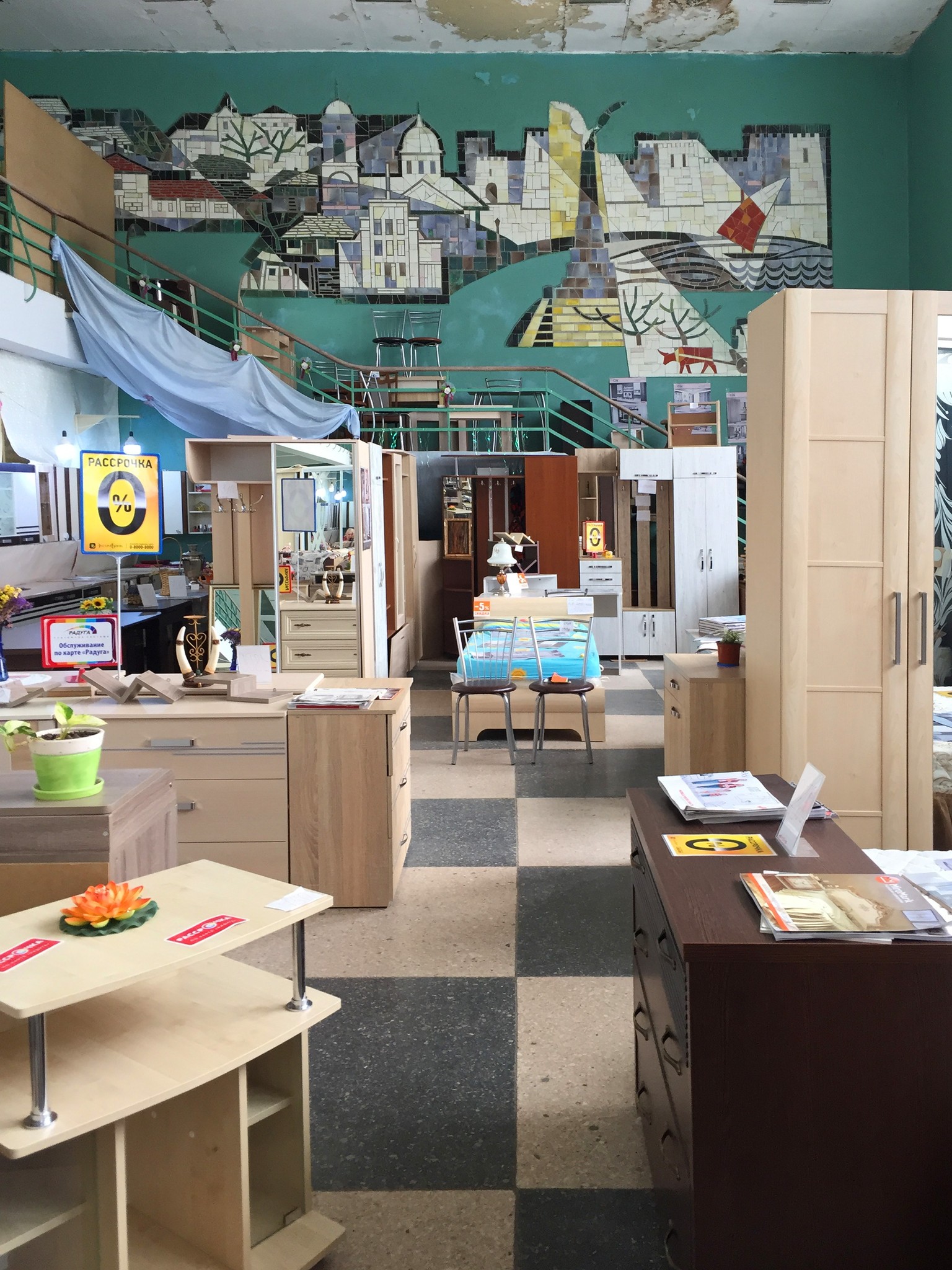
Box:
[717,628,743,665]
[1,700,107,801]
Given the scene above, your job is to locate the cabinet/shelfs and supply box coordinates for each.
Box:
[624,772,952,1268]
[576,448,739,657]
[744,286,950,851]
[1,858,348,1269]
[239,324,297,390]
[0,434,423,914]
[663,652,745,778]
[440,474,524,656]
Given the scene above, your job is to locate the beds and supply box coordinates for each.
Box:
[451,596,606,742]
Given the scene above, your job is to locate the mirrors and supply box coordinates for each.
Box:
[271,441,357,604]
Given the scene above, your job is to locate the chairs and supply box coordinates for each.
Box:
[528,616,595,766]
[301,307,524,452]
[451,616,517,766]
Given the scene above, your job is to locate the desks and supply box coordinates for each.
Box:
[467,391,551,452]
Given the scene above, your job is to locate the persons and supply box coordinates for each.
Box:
[343,529,354,541]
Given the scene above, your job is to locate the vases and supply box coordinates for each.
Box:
[0,624,9,682]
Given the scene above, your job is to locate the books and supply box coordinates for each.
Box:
[698,615,746,635]
[657,771,840,823]
[739,869,952,945]
[287,685,402,710]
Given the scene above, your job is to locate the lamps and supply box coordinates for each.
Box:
[486,537,517,594]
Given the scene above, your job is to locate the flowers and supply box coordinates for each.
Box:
[0,585,34,628]
[77,595,113,615]
